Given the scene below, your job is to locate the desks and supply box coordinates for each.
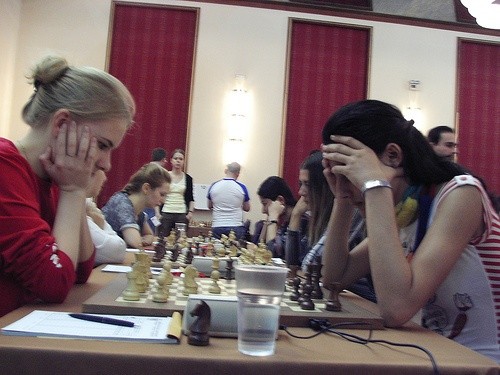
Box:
[0,253,500,375]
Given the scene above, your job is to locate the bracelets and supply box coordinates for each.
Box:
[267,220,277,225]
[362,179,393,198]
[187,210,194,213]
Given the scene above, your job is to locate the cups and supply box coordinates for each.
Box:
[237,265,292,358]
[175,223,186,235]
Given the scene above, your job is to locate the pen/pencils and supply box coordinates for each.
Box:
[69,313,135,328]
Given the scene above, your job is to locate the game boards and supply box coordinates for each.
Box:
[82,241,387,330]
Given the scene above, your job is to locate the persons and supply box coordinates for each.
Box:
[257,176,313,269]
[319,100,500,366]
[206,162,251,243]
[101,161,171,248]
[144,147,168,236]
[427,126,457,163]
[154,149,194,239]
[0,55,136,317]
[285,152,377,302]
